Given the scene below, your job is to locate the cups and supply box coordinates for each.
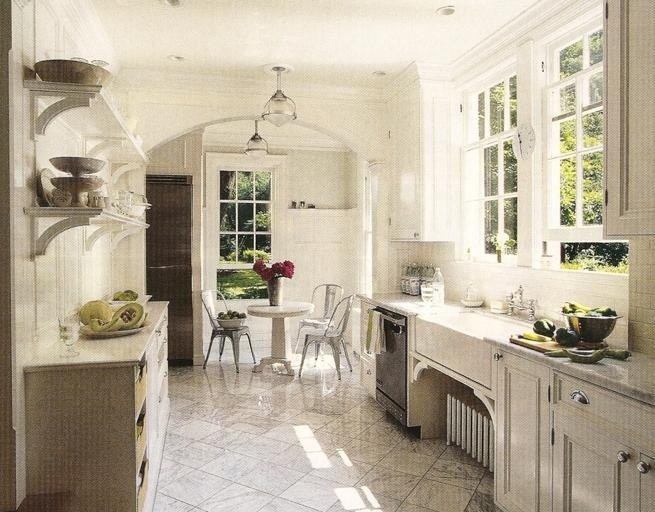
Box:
[419,283,434,304]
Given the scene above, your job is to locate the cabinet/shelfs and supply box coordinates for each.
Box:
[551,368,655,512]
[602,2,653,237]
[25,301,168,512]
[387,80,462,242]
[490,347,549,511]
[360,300,497,441]
[26,79,151,255]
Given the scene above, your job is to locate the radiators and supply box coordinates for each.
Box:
[446,391,494,473]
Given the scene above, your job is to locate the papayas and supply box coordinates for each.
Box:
[80,300,144,332]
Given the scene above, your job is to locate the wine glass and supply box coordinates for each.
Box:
[48,155,107,178]
[48,173,107,208]
[57,316,83,359]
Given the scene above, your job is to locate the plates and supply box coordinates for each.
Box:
[107,293,152,312]
[77,321,154,340]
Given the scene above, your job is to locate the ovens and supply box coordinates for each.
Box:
[365,305,410,413]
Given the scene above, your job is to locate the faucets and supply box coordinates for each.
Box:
[512,284,523,308]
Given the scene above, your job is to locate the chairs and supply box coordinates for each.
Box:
[200,283,352,380]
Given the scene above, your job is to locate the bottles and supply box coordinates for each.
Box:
[398,263,436,296]
[432,266,446,308]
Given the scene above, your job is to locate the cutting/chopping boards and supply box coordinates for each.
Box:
[509,333,613,358]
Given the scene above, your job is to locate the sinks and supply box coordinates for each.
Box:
[414,309,537,390]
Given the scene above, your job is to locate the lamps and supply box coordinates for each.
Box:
[242,119,268,159]
[261,62,297,126]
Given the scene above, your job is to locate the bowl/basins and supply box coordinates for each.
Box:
[560,309,624,350]
[213,317,250,331]
[460,298,485,308]
[33,58,113,88]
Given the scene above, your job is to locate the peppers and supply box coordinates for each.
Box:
[555,328,580,347]
[543,348,578,357]
[533,319,556,337]
[522,331,553,342]
[563,349,607,363]
[605,350,632,359]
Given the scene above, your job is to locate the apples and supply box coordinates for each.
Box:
[218,310,246,319]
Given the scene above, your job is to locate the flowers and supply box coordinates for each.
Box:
[253,259,295,283]
